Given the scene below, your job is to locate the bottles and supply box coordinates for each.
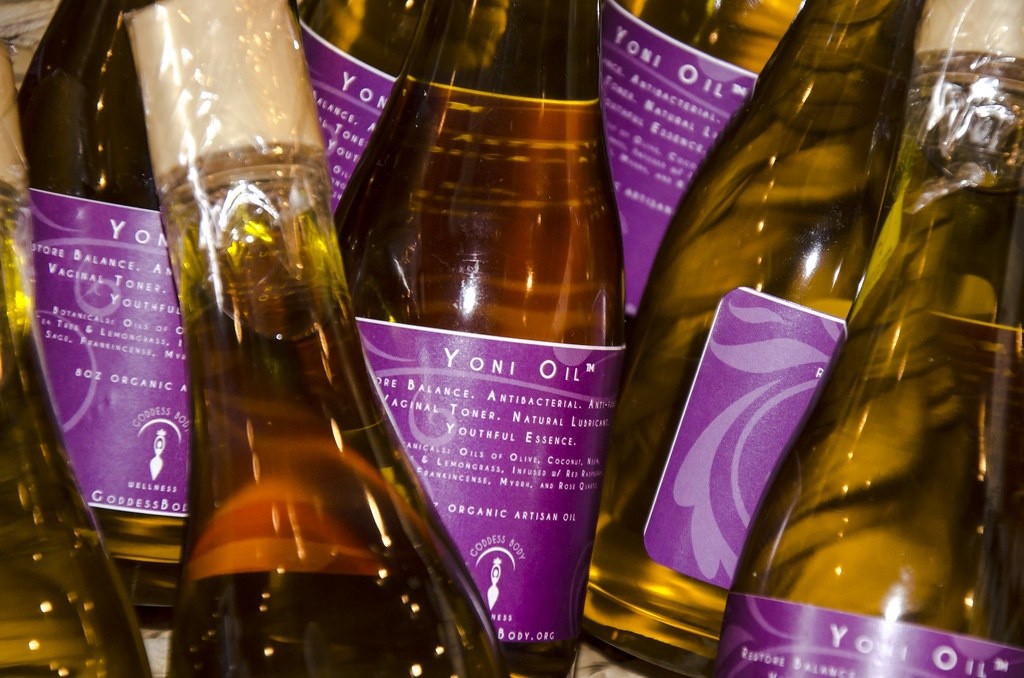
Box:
[0,0,1024,678]
